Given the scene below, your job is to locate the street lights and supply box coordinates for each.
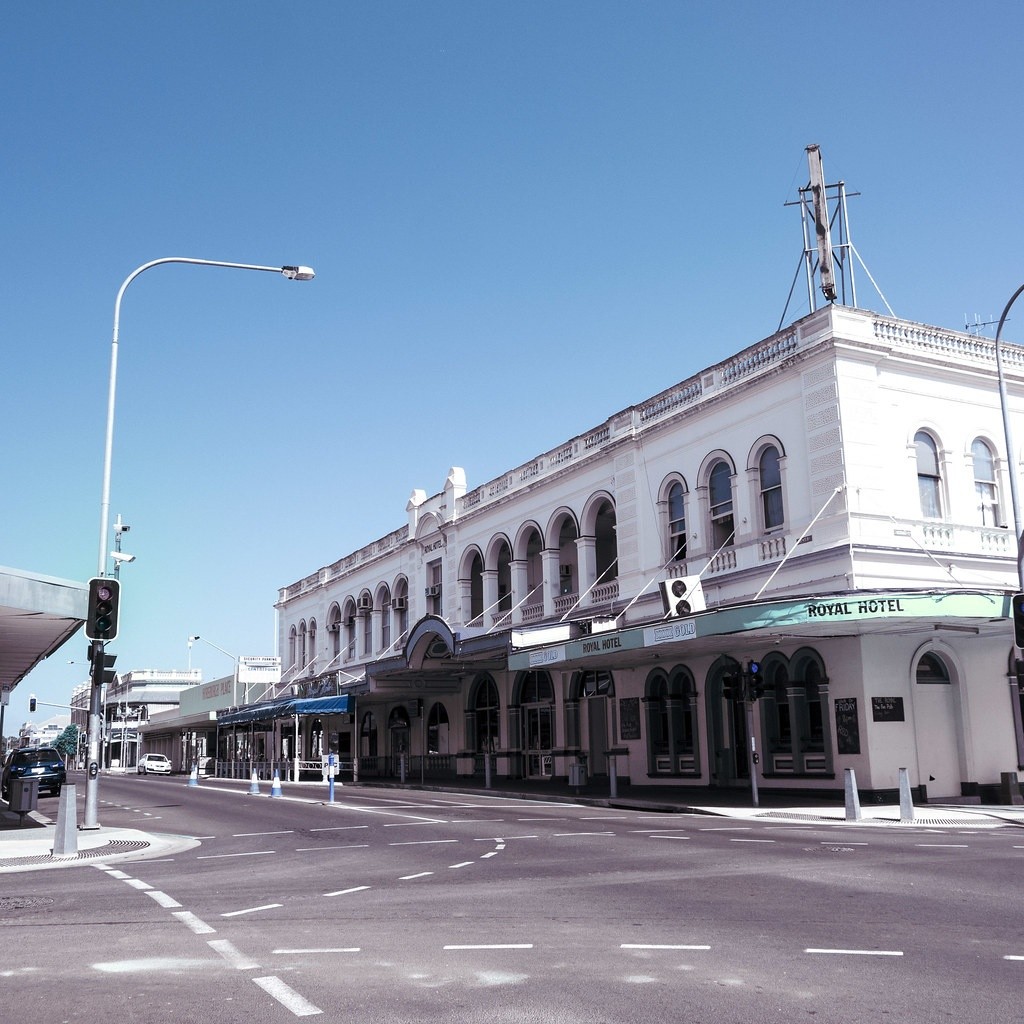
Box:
[189,635,238,707]
[82,256,317,823]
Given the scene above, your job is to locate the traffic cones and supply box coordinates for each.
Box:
[248,768,262,795]
[270,768,283,797]
[188,763,199,788]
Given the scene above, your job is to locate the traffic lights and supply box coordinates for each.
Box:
[747,660,764,700]
[722,673,747,701]
[1012,593,1024,649]
[96,652,117,684]
[86,577,118,641]
[30,699,37,712]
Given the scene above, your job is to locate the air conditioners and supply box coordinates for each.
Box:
[658,575,707,619]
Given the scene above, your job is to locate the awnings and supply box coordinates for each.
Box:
[0,565,89,709]
[215,694,352,729]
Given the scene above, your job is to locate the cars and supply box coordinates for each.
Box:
[138,752,172,775]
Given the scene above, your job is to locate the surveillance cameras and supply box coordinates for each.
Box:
[110,524,136,563]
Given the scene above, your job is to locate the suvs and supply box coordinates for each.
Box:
[3,746,66,799]
